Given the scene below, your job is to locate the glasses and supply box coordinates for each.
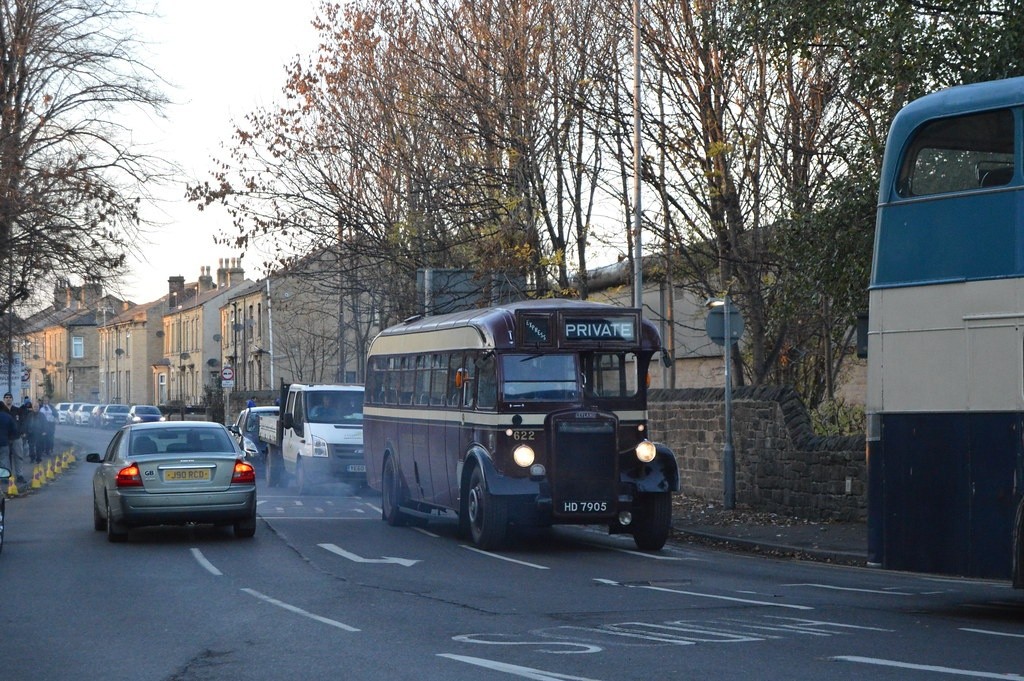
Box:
[4,397,12,399]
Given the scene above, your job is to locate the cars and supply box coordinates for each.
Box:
[232,406,281,476]
[125,405,165,424]
[85,420,258,544]
[55,402,131,429]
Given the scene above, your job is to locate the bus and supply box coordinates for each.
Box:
[362,298,680,551]
[863,75,1024,592]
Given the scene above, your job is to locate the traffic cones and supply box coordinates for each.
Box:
[7,474,19,496]
[32,447,77,490]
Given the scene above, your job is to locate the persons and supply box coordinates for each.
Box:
[310,394,335,416]
[247,396,257,408]
[0,392,59,484]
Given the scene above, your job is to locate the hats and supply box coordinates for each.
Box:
[24,396,32,408]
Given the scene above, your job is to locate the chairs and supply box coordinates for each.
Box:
[167,443,191,452]
[564,370,587,399]
[137,409,156,413]
[201,439,219,451]
[310,404,324,417]
[977,159,1014,186]
[378,390,474,407]
[108,408,129,413]
[134,437,158,453]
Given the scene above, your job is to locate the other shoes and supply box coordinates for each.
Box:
[30,459,35,463]
[37,458,41,462]
[17,476,27,484]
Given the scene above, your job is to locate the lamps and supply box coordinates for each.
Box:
[194,287,198,301]
[173,291,178,310]
[161,299,166,315]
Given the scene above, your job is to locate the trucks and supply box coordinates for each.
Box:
[258,377,369,495]
